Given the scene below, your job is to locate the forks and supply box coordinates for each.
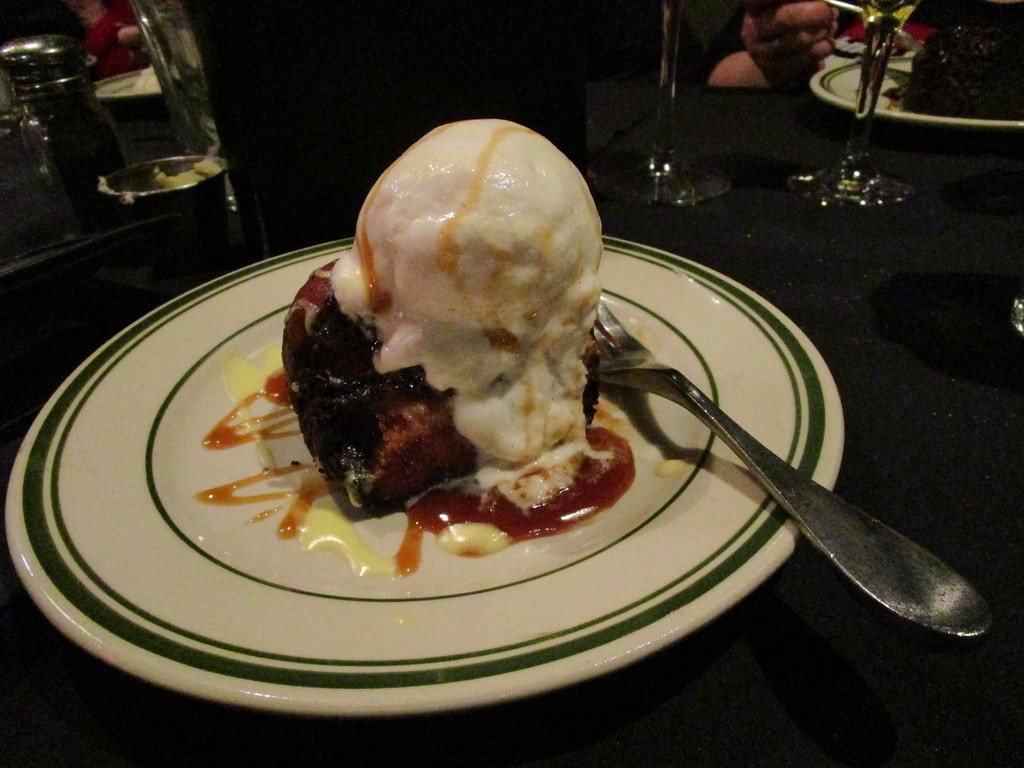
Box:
[584,297,994,638]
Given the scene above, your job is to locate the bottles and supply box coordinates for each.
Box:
[1,33,128,234]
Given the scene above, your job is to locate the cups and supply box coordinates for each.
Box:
[129,0,222,156]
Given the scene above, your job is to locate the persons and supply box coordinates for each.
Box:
[703,0,1024,89]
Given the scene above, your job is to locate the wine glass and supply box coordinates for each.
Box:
[784,0,934,214]
[595,0,734,210]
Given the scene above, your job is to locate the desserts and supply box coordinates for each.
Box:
[281,118,606,519]
[900,25,1024,123]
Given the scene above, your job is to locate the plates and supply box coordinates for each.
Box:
[808,54,1024,132]
[91,71,163,100]
[6,234,847,719]
[97,155,227,206]
